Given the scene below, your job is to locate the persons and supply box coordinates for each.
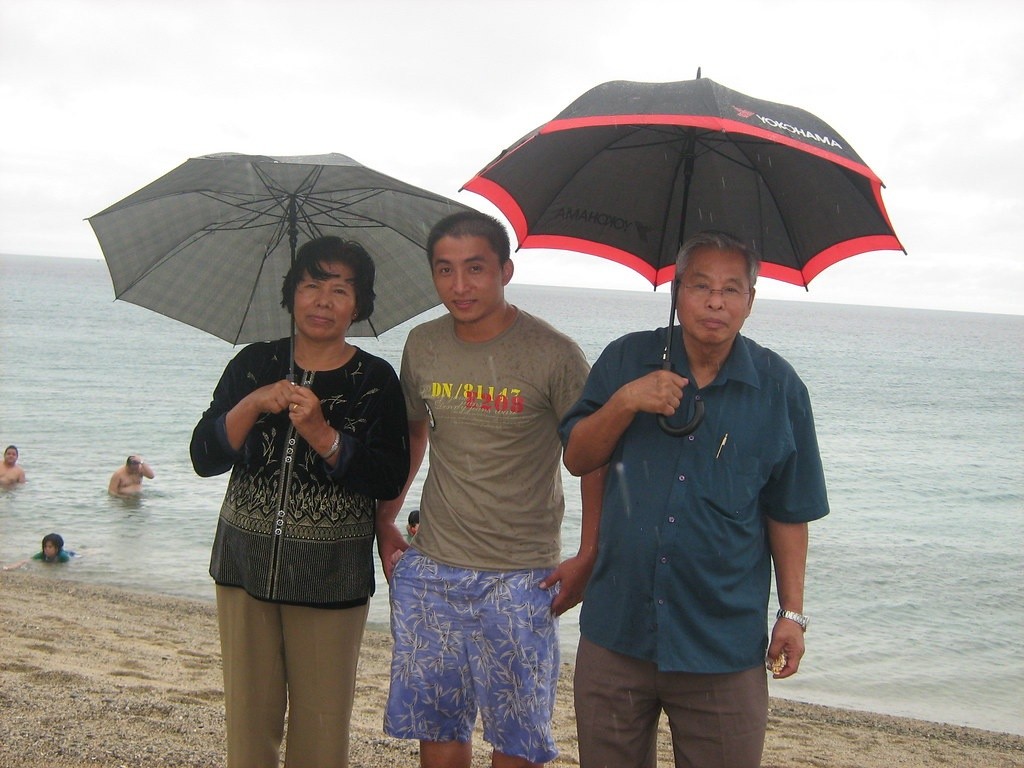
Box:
[404,509,421,537]
[555,231,831,768]
[2,533,76,571]
[188,237,412,768]
[374,211,606,768]
[0,445,25,488]
[108,456,154,500]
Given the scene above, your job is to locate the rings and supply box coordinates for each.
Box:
[292,404,298,412]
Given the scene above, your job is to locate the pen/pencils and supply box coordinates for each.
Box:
[715,433,729,459]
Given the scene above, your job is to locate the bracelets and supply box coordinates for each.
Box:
[776,609,810,631]
[140,461,144,465]
[320,430,341,460]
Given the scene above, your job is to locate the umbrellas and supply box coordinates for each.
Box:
[81,151,482,386]
[457,67,909,438]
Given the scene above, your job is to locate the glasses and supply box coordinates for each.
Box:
[131,461,138,465]
[678,280,753,298]
